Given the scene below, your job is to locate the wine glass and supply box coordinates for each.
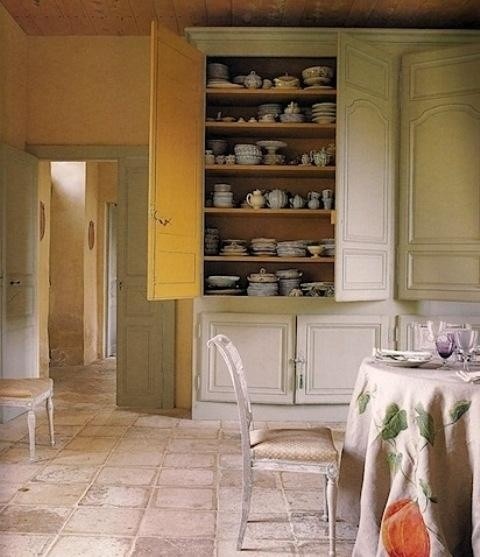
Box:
[428,322,476,373]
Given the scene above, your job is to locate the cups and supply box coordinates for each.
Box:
[301,154,311,165]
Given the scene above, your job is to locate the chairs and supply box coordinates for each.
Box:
[203,334,340,556]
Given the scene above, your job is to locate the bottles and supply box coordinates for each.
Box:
[307,188,332,210]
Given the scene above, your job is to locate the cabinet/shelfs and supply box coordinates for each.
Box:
[146,18,480,419]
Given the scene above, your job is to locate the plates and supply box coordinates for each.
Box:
[246,279,334,297]
[211,184,234,209]
[205,289,244,295]
[258,103,336,124]
[218,238,335,257]
[377,352,433,368]
[208,63,243,88]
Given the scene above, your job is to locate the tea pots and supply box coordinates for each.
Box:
[284,100,301,114]
[245,190,304,209]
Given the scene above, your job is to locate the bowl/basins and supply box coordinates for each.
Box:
[234,142,287,164]
[207,140,226,154]
[303,65,333,89]
[206,275,240,289]
[204,228,220,255]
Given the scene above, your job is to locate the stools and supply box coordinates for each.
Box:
[1,379,56,457]
